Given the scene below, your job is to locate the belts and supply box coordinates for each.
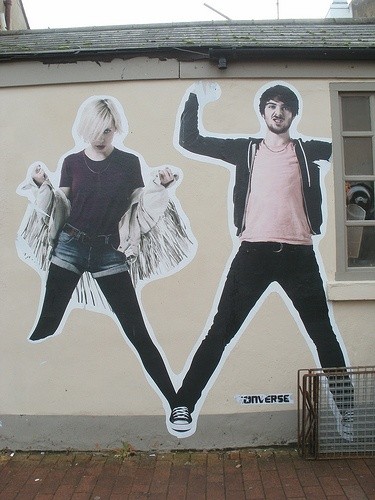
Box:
[250,242,307,252]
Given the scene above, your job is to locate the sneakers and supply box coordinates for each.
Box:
[341,411,353,428]
[169,406,192,431]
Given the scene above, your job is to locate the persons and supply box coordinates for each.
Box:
[29,97,177,412]
[169,84,358,440]
